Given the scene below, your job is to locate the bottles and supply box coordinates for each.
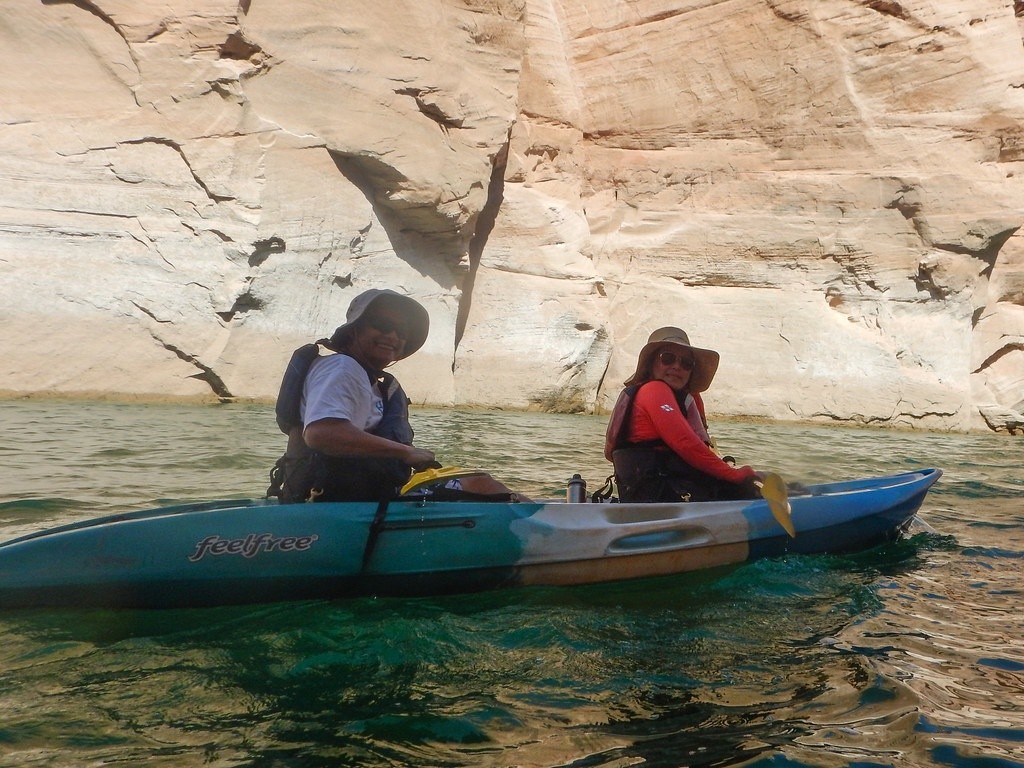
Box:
[567,473,586,504]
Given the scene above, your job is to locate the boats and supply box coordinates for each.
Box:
[0,462,943,614]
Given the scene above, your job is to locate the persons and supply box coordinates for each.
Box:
[604,326,758,502]
[274,288,435,501]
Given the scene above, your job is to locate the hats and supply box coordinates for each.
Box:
[623,326,720,396]
[329,288,430,362]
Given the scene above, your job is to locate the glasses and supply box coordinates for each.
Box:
[656,351,696,372]
[357,317,413,343]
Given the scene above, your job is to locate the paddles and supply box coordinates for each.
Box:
[708,436,797,539]
[398,459,489,499]
